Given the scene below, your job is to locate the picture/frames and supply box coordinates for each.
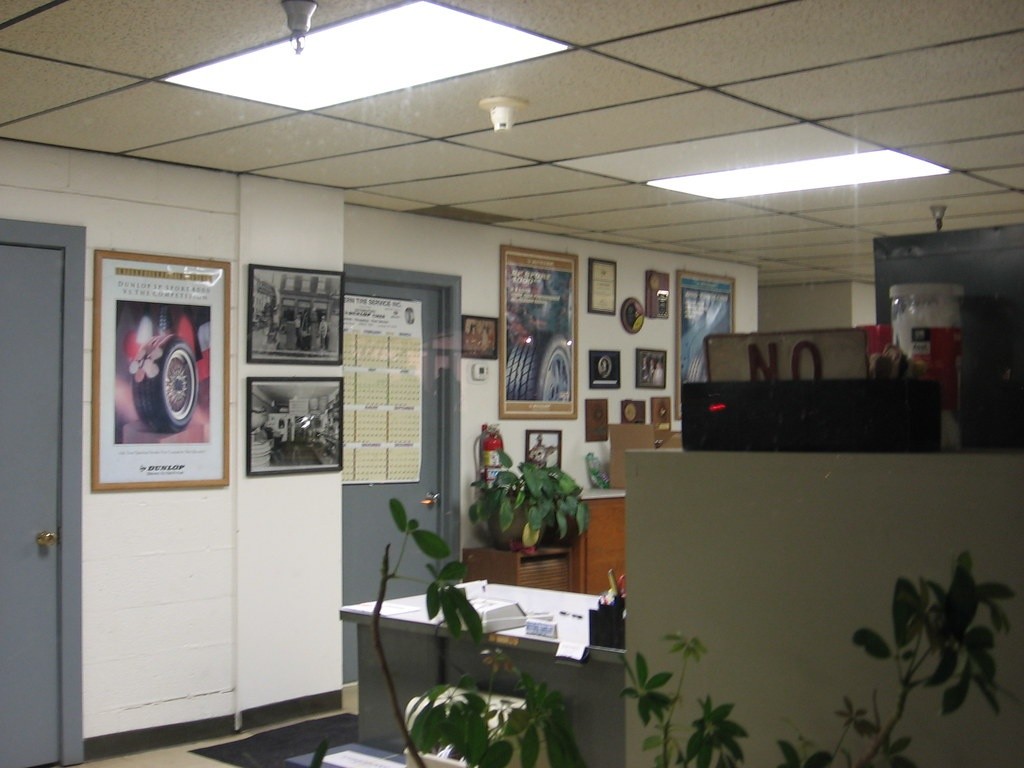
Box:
[245,376,344,475]
[246,263,345,367]
[636,348,667,390]
[674,269,736,419]
[589,349,622,390]
[90,249,230,493]
[461,314,498,361]
[499,244,579,420]
[526,429,561,470]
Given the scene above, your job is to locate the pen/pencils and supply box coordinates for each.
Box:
[557,610,586,619]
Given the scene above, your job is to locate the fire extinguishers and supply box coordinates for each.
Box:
[479,420,503,487]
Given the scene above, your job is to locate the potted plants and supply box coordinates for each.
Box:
[469,448,589,551]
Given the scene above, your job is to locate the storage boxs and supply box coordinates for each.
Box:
[609,423,683,489]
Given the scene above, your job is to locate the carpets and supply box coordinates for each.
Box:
[338,583,628,768]
[188,712,358,768]
